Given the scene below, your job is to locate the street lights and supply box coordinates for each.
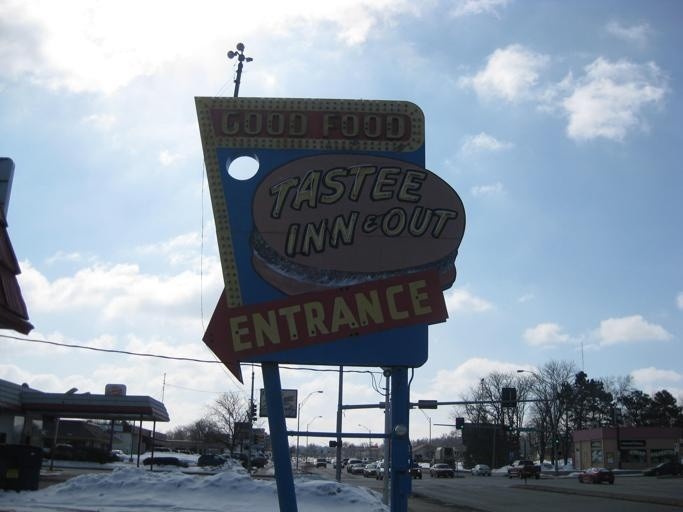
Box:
[516,370,558,475]
[305,415,321,464]
[296,390,322,471]
[357,423,370,461]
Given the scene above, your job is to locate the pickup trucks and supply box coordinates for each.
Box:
[507,459,541,478]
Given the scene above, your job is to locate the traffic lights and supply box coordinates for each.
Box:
[555,434,558,444]
[507,421,513,432]
[252,403,256,421]
[455,418,464,430]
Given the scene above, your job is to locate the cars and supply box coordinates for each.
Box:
[410,461,422,479]
[363,464,377,478]
[375,461,391,479]
[428,462,453,478]
[578,467,615,483]
[641,462,682,477]
[471,463,491,476]
